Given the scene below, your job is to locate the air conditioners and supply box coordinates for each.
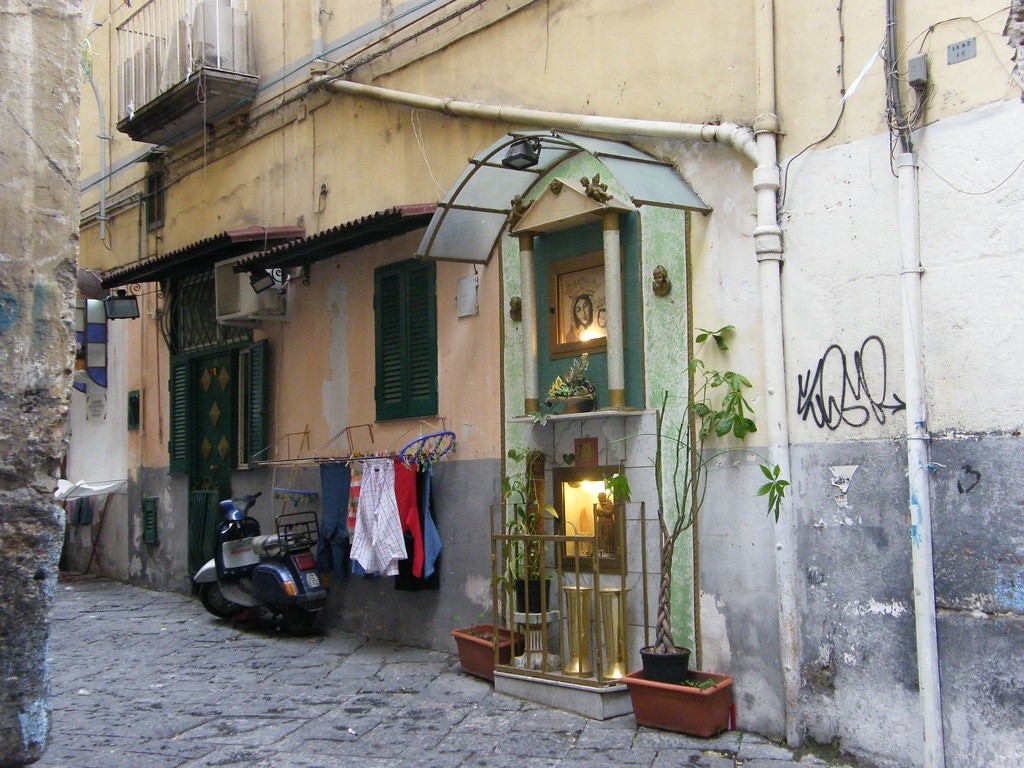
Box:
[212,253,289,326]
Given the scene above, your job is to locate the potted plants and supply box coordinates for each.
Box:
[638,325,784,687]
[492,448,553,612]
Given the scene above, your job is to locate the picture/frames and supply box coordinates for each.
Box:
[548,252,626,355]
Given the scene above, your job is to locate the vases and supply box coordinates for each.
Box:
[449,617,524,680]
[618,668,736,736]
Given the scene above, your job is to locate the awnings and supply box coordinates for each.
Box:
[98,224,306,290]
[414,129,711,264]
[232,201,437,275]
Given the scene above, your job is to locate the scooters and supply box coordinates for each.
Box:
[193,488,330,636]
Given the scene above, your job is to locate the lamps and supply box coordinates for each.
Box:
[246,259,276,295]
[105,290,139,319]
